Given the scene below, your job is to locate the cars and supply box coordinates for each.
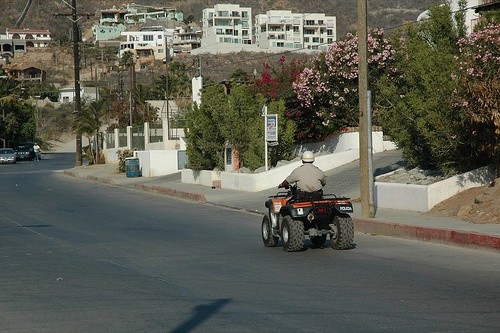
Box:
[15,142,38,161]
[0,148,16,164]
[38,151,42,161]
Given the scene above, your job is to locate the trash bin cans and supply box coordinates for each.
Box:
[125,157,140,178]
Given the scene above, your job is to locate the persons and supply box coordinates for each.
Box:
[278,151,326,200]
[33,142,40,161]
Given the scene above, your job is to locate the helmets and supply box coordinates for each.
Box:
[301,151,315,162]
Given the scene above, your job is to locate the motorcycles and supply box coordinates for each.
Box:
[262,185,354,252]
